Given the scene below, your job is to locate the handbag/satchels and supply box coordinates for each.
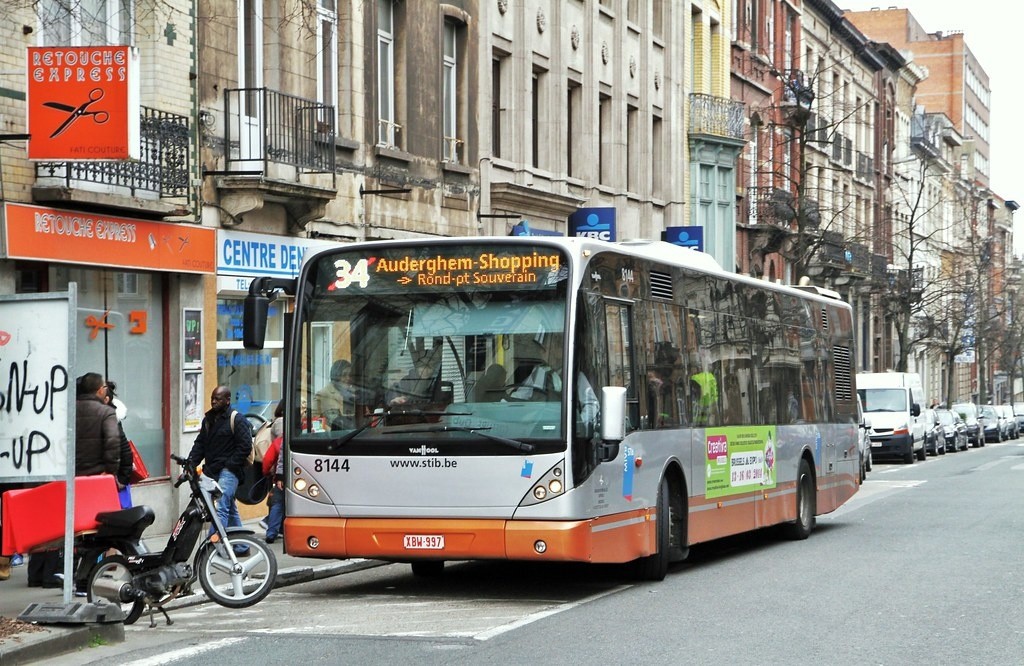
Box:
[236,461,269,505]
[128,440,149,485]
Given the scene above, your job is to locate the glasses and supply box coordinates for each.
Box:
[103,385,108,390]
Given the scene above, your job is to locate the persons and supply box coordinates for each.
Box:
[500,333,601,437]
[310,352,370,430]
[467,364,508,403]
[383,349,438,407]
[183,386,284,557]
[0,373,145,597]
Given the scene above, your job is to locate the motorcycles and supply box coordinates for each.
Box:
[41,455,278,626]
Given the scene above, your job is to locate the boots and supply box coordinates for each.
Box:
[0,557,11,579]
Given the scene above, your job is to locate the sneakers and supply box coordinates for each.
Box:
[11,556,24,566]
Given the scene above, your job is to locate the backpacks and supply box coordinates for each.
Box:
[205,410,255,465]
[254,419,274,463]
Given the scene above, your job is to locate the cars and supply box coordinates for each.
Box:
[925,403,1024,456]
[856,392,872,483]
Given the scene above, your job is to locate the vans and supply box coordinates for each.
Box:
[858,371,928,463]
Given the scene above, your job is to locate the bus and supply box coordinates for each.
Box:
[243,237,860,580]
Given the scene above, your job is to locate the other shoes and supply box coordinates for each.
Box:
[75,584,88,596]
[276,529,283,537]
[43,581,62,588]
[28,580,42,587]
[266,536,275,544]
[217,547,250,558]
[258,518,270,530]
